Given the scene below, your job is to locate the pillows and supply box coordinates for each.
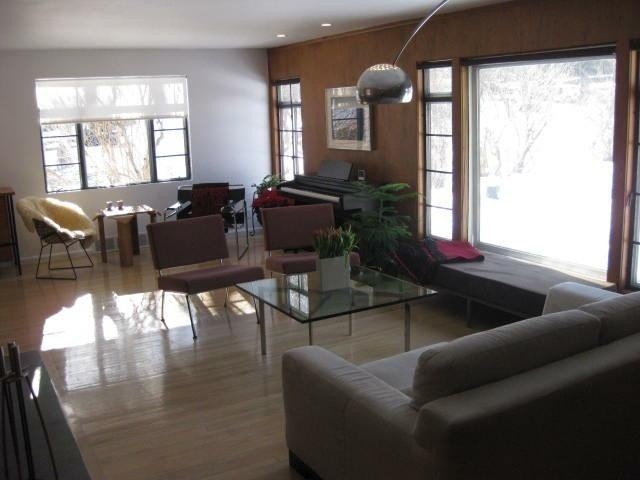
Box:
[413,310,602,410]
[581,290,640,348]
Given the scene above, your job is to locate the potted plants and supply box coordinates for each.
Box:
[252,174,286,198]
[350,179,425,271]
[311,227,354,293]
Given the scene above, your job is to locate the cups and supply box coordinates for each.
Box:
[105,201,113,211]
[116,200,124,210]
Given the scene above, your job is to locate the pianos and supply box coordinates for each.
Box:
[277,159,382,212]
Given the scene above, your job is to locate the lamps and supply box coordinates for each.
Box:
[356,1,452,107]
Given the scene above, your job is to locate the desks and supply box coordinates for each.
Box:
[100,203,158,267]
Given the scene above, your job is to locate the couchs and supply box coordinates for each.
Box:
[282,280,639,478]
[385,235,640,326]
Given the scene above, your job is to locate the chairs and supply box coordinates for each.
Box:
[147,214,266,341]
[17,196,97,282]
[259,204,363,321]
[177,183,249,261]
[255,200,300,254]
